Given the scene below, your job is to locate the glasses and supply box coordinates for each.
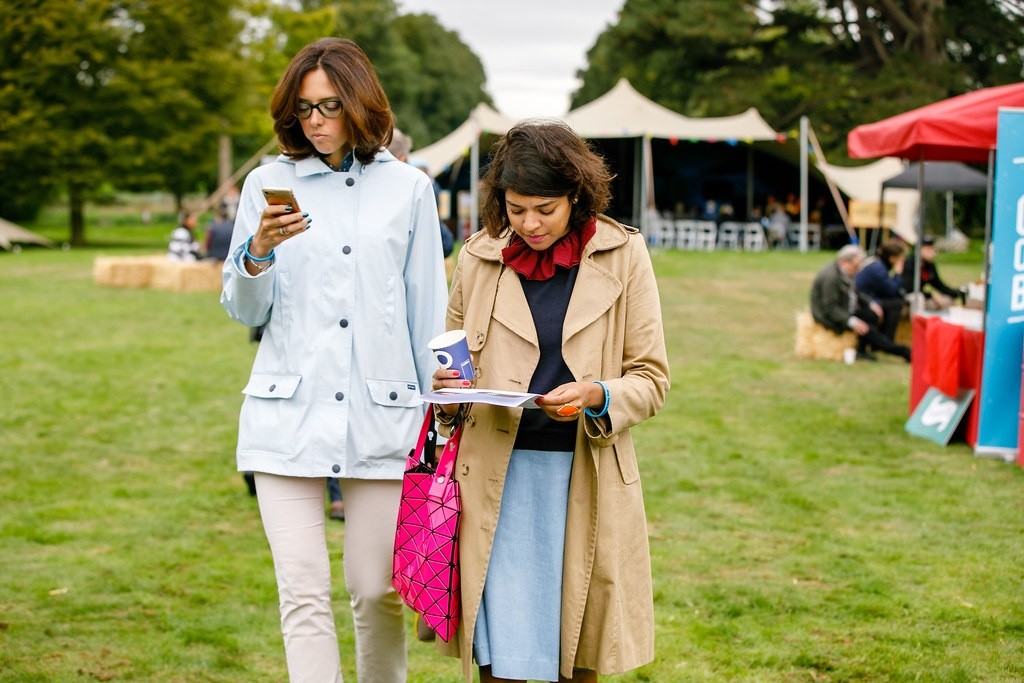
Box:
[293,100,344,120]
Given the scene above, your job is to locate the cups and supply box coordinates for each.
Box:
[428,329,474,381]
[844,349,856,364]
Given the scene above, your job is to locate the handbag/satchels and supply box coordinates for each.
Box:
[392,403,463,643]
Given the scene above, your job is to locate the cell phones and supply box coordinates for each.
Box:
[262,188,300,218]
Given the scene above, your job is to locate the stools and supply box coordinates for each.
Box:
[793,314,857,361]
[92,255,219,292]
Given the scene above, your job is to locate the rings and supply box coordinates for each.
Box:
[280,228,290,236]
[556,403,579,417]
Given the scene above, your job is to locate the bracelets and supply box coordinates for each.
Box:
[586,381,611,417]
[245,234,275,271]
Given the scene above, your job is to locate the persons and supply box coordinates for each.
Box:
[431,125,670,683]
[220,37,450,683]
[167,128,968,364]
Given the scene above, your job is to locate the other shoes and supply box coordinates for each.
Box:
[857,349,878,362]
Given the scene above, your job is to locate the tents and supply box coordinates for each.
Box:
[848,82,1024,468]
[402,79,988,254]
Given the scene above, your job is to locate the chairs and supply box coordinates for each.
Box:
[654,219,821,251]
